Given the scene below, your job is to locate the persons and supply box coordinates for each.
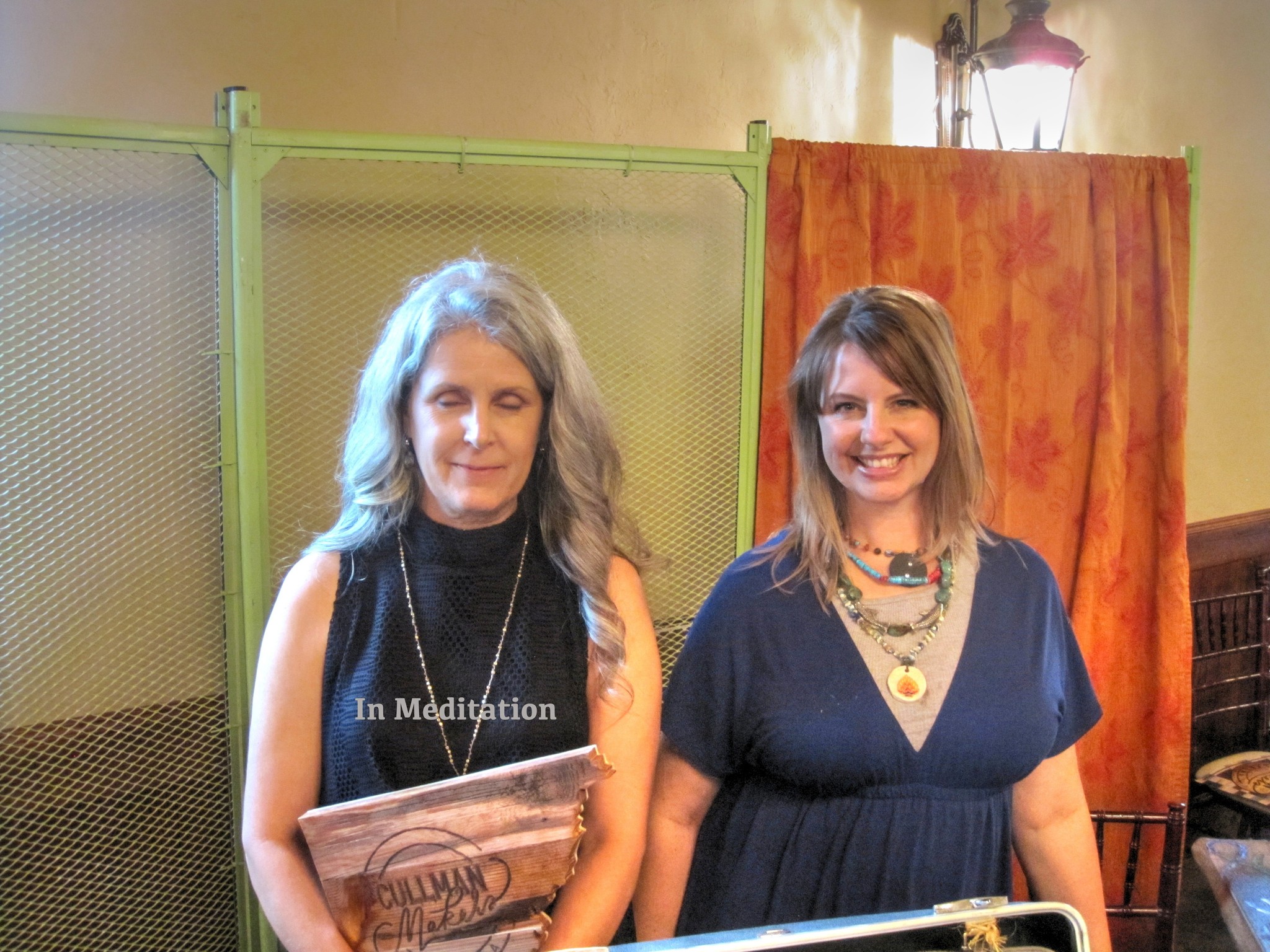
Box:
[598,281,1124,952]
[233,254,668,951]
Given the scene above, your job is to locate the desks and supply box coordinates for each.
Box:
[1189,837,1270,952]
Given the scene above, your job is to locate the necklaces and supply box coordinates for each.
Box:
[396,488,532,779]
[818,509,960,704]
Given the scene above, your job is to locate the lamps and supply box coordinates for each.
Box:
[934,0,1092,154]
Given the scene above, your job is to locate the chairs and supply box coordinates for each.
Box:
[1185,564,1270,854]
[1087,800,1186,952]
